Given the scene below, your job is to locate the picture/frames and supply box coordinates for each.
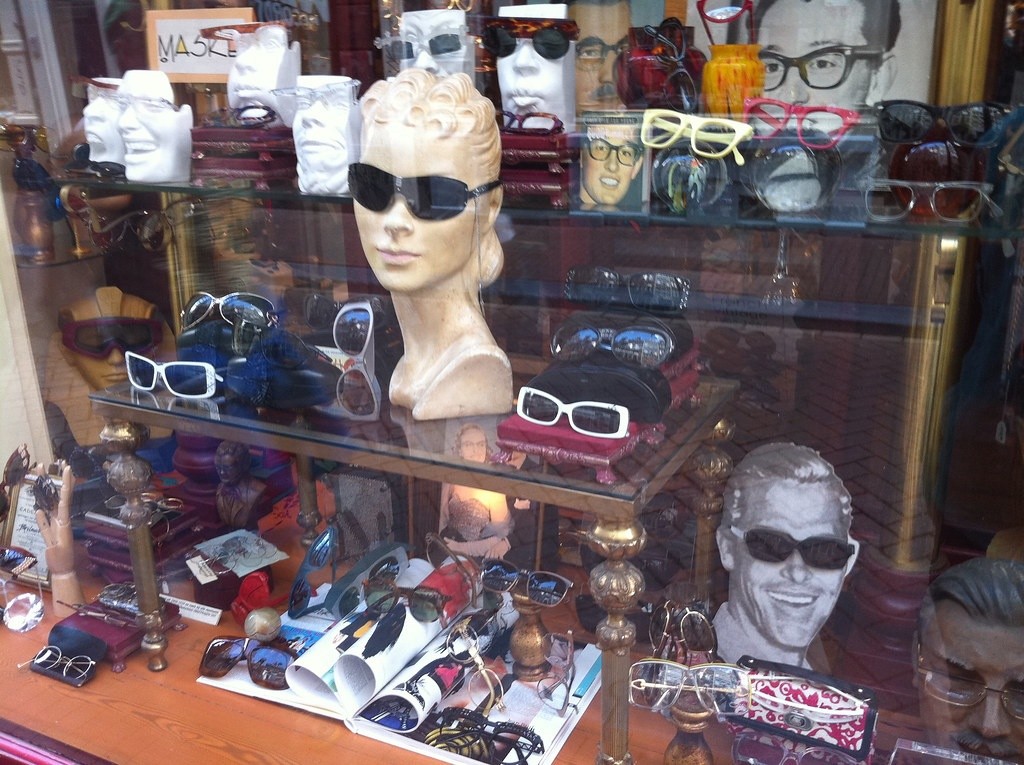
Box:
[1,472,64,594]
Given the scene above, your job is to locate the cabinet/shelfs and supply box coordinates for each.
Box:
[0,74,1024,765]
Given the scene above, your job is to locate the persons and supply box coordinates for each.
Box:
[438,415,541,569]
[576,119,647,214]
[747,1,902,134]
[82,76,129,178]
[490,3,575,130]
[59,285,163,393]
[114,70,194,183]
[574,0,635,114]
[712,442,860,672]
[910,554,1023,765]
[226,25,302,131]
[387,10,477,90]
[34,460,87,617]
[347,68,516,421]
[215,440,269,531]
[292,74,361,198]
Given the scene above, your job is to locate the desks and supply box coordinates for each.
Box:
[89,352,744,765]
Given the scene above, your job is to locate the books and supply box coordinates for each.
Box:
[198,551,602,765]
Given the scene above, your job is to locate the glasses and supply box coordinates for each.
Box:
[0,440,878,765]
[585,136,643,167]
[516,385,630,439]
[563,263,690,316]
[62,317,163,358]
[385,32,468,59]
[100,195,217,255]
[202,105,275,130]
[727,523,855,570]
[549,321,673,370]
[482,20,570,59]
[346,161,502,221]
[758,44,884,91]
[124,290,382,422]
[645,15,701,113]
[69,73,119,99]
[915,641,1024,726]
[637,94,1009,225]
[493,109,564,136]
[0,123,60,155]
[65,157,126,179]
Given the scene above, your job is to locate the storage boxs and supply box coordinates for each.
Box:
[191,126,298,189]
[500,134,579,209]
